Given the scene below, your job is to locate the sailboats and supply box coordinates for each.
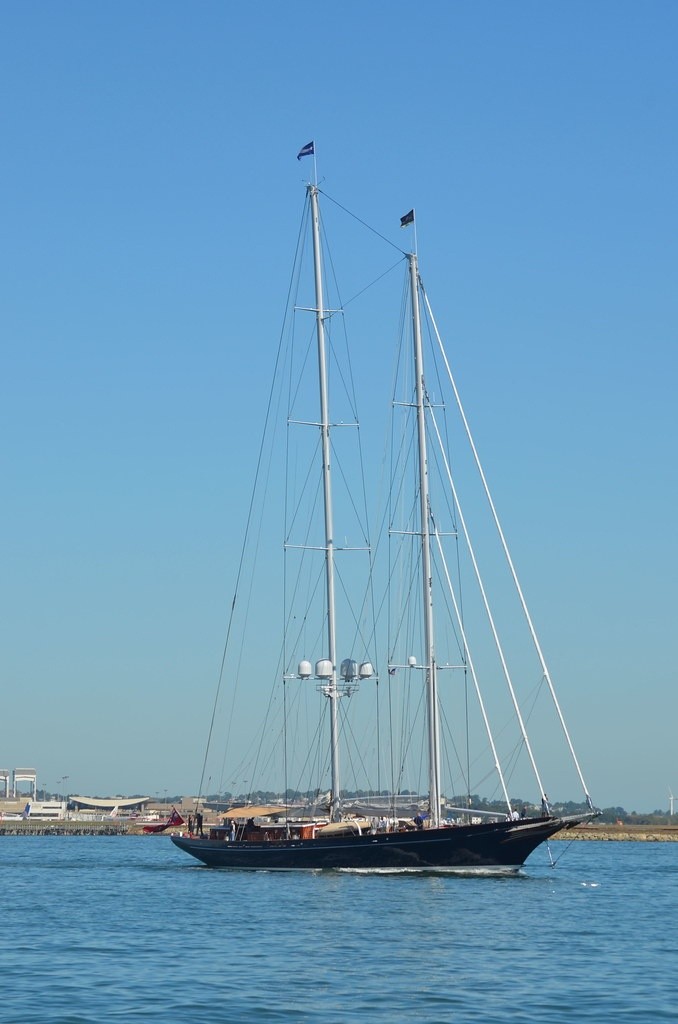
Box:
[168,134,602,869]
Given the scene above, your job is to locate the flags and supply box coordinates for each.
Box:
[142,808,185,833]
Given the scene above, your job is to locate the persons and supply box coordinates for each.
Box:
[229,820,236,842]
[512,808,520,821]
[521,805,529,821]
[196,809,204,836]
[544,793,549,817]
[541,798,546,818]
[188,814,196,840]
[380,816,386,832]
[414,812,424,831]
[371,817,377,832]
[505,812,512,822]
